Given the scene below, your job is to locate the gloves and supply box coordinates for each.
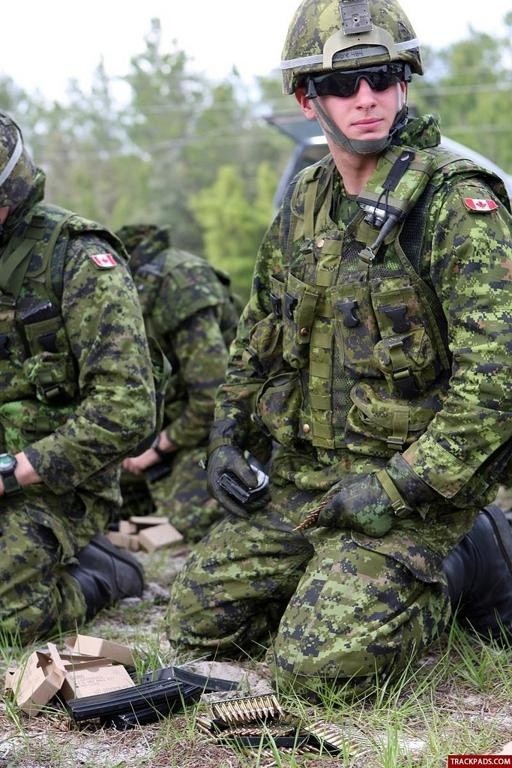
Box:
[317,453,435,538]
[206,421,258,519]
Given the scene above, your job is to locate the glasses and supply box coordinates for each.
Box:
[304,62,411,97]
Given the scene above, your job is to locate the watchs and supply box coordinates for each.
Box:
[2,455,23,494]
[152,434,172,462]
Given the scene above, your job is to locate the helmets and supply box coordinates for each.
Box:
[280,0,423,95]
[0,109,35,203]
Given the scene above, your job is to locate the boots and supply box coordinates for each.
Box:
[442,504,511,649]
[69,537,144,623]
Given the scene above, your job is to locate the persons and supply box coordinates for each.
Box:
[111,224,243,538]
[167,0,512,709]
[1,112,156,644]
